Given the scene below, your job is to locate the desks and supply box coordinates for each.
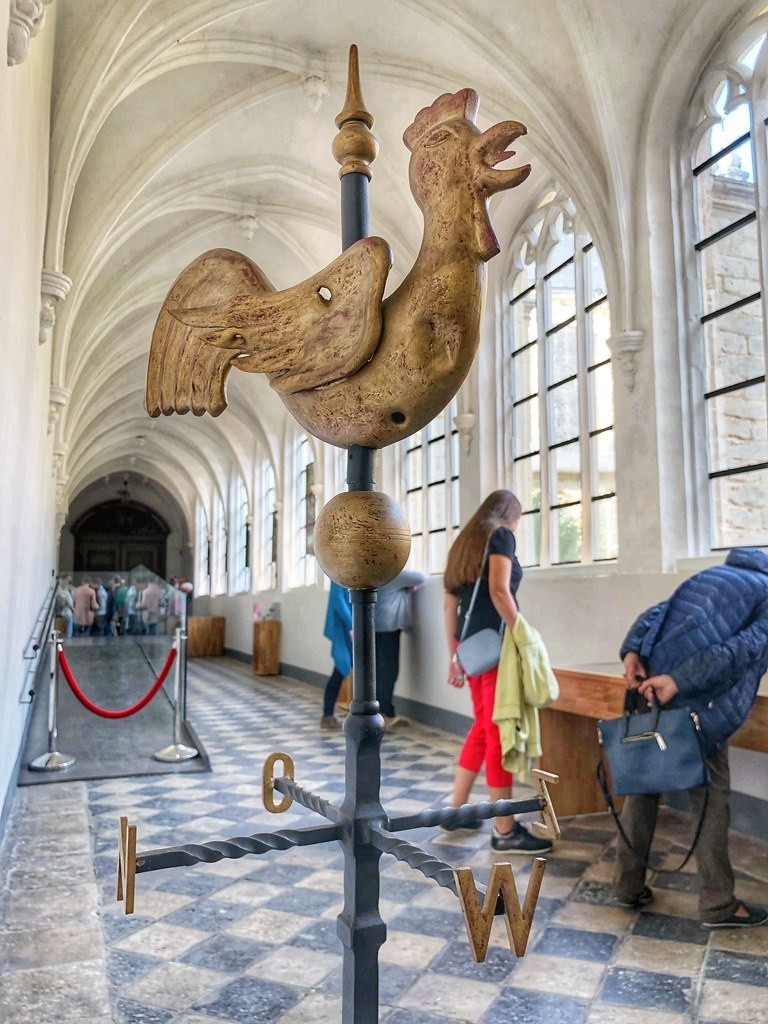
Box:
[537,660,768,824]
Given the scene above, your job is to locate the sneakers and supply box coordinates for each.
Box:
[440,818,483,835]
[490,821,553,854]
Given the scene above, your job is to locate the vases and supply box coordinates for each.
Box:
[252,619,280,674]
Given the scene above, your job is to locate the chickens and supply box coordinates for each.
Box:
[142,84,532,453]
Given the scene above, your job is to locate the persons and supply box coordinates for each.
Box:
[321,580,353,732]
[56,570,192,638]
[443,489,554,855]
[375,568,427,727]
[613,566,767,931]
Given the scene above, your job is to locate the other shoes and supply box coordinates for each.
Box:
[319,715,343,732]
[384,716,408,726]
[701,900,768,928]
[619,887,654,908]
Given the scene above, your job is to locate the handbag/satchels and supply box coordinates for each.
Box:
[456,628,503,676]
[596,676,712,798]
